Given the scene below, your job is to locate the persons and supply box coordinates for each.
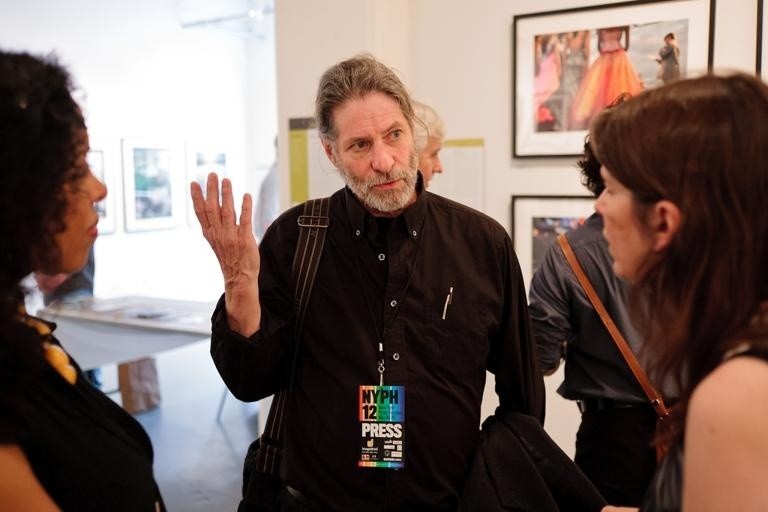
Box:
[588,65,766,512]
[533,34,572,132]
[651,31,682,85]
[406,97,445,192]
[188,52,547,511]
[0,43,168,510]
[523,133,691,511]
[570,24,644,132]
[558,31,593,132]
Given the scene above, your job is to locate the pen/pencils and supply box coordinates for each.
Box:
[443,287,456,319]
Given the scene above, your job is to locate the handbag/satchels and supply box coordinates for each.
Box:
[238,435,280,511]
[656,402,685,464]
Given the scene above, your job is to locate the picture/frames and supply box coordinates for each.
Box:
[121,134,184,230]
[513,0,721,158]
[511,193,596,305]
[85,147,115,236]
